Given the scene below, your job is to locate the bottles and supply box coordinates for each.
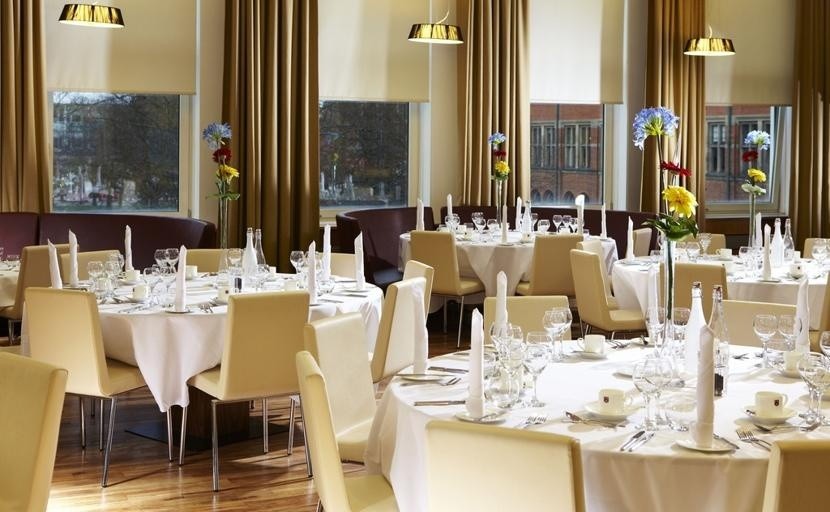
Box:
[685,282,708,362]
[784,219,795,261]
[771,217,784,264]
[242,227,258,274]
[522,201,531,232]
[708,284,730,366]
[255,228,266,274]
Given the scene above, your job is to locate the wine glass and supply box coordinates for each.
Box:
[0,246,20,271]
[88,248,334,297]
[522,345,551,407]
[542,310,567,363]
[525,331,550,347]
[806,356,829,428]
[644,308,666,354]
[796,353,826,421]
[642,361,676,427]
[550,307,574,360]
[650,233,829,276]
[444,212,577,244]
[671,308,690,351]
[500,343,525,406]
[775,315,803,364]
[633,363,663,429]
[753,315,779,368]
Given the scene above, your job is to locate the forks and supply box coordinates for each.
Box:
[737,432,770,453]
[526,416,547,428]
[745,431,773,447]
[521,417,537,428]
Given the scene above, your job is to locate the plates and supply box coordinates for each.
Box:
[165,307,191,313]
[742,406,794,425]
[455,410,505,425]
[584,401,642,420]
[672,429,736,453]
[572,348,616,359]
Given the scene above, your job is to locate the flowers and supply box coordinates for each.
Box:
[738,127,770,232]
[632,104,698,318]
[201,120,241,245]
[487,132,510,223]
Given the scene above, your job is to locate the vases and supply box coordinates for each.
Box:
[217,197,230,249]
[495,179,503,229]
[744,192,762,269]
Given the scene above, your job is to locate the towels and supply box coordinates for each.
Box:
[502,204,508,243]
[516,196,523,215]
[446,193,454,216]
[415,198,426,228]
[574,194,585,220]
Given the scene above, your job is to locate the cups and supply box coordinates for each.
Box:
[597,389,633,411]
[489,321,512,349]
[788,262,805,279]
[818,330,830,357]
[576,334,606,355]
[500,325,524,349]
[753,391,789,415]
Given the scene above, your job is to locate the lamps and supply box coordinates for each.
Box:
[59,0,126,30]
[682,23,735,57]
[407,0,464,44]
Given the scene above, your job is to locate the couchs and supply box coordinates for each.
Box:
[439,205,660,258]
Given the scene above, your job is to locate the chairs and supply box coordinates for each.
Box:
[334,205,436,290]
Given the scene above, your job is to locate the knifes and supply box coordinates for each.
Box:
[630,433,654,450]
[621,431,644,449]
